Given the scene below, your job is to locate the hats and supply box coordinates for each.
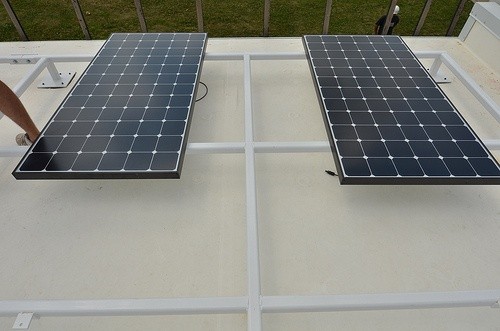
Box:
[392,5,400,14]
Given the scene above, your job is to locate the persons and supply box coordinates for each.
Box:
[0,81,40,147]
[374,5,400,35]
